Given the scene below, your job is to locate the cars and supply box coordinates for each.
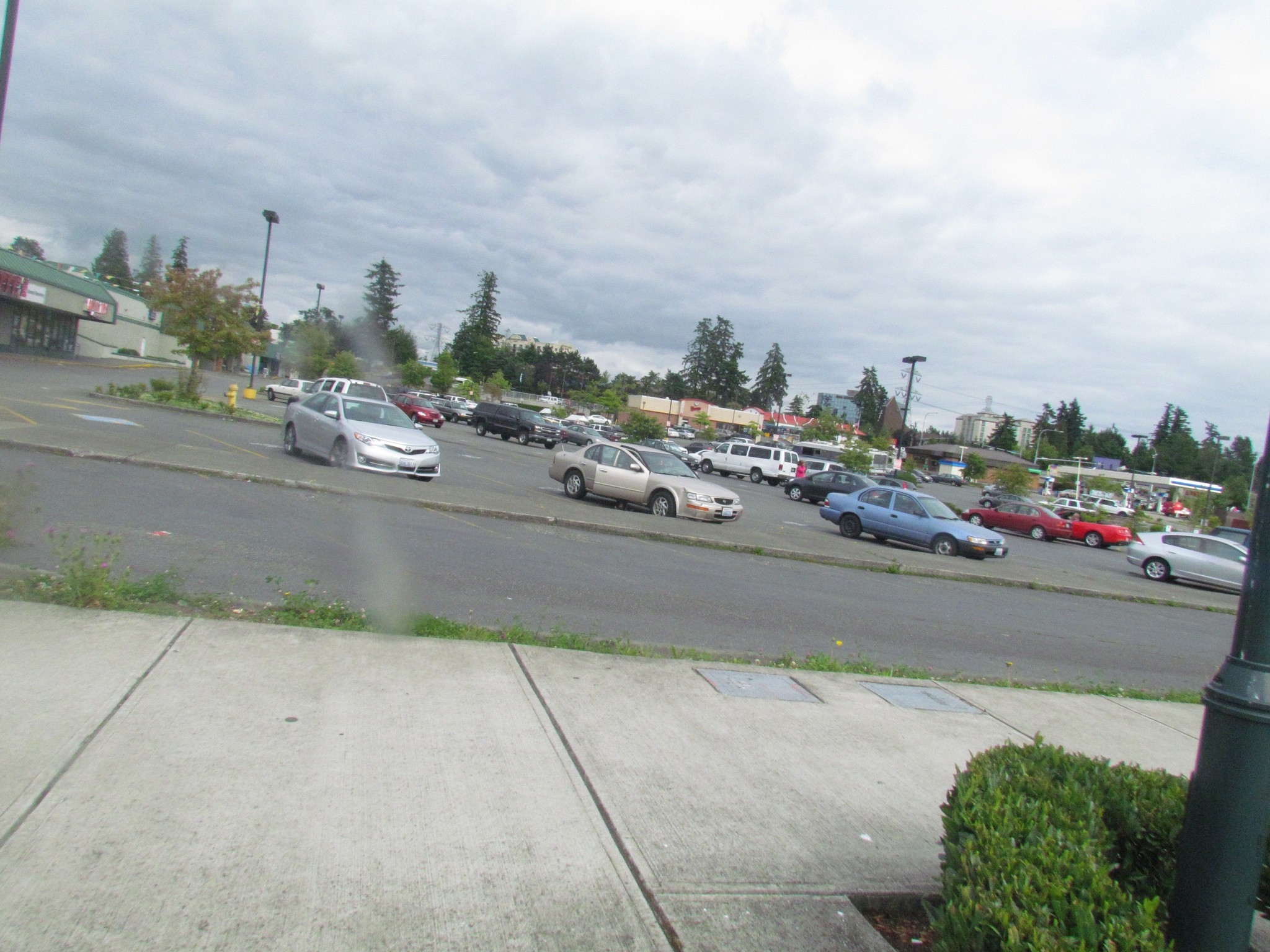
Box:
[979,484,1036,509]
[283,390,441,482]
[542,413,967,504]
[1038,489,1135,517]
[263,378,315,401]
[383,388,475,428]
[1126,530,1249,593]
[961,501,1073,542]
[548,441,744,524]
[819,486,1010,560]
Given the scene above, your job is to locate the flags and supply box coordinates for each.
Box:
[520,373,522,382]
[55,263,142,293]
[7,246,38,260]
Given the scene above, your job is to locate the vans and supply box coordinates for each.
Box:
[539,396,558,405]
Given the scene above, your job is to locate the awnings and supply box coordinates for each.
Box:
[765,423,774,427]
[780,426,798,431]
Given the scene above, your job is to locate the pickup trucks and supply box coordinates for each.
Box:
[1160,502,1191,518]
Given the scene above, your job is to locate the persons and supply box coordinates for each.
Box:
[1079,516,1085,522]
[796,461,806,477]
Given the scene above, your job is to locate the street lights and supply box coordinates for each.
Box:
[1200,432,1231,535]
[1034,429,1064,464]
[775,373,792,434]
[338,314,344,336]
[248,210,281,389]
[892,355,928,479]
[1129,434,1149,489]
[305,282,326,379]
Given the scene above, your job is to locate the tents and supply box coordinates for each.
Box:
[565,414,589,424]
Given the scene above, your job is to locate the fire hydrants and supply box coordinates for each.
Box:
[223,384,239,409]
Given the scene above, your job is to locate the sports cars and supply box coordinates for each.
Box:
[1056,511,1133,549]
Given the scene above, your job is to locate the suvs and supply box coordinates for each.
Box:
[471,401,568,449]
[1200,527,1252,561]
[287,378,388,419]
